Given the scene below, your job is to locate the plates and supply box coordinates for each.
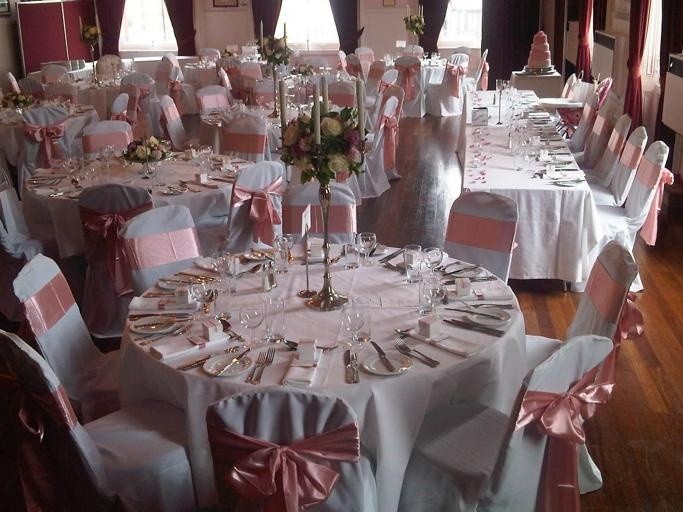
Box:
[520,90,585,188]
[127,243,513,379]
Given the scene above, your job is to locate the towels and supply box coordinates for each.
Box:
[150,319,228,359]
[405,314,483,358]
[281,340,324,387]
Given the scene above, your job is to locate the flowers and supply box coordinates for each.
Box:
[258,35,295,66]
[78,23,100,42]
[276,99,370,186]
[401,4,426,35]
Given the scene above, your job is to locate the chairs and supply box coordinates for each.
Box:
[1,329,195,512]
[0,45,674,338]
[8,252,128,421]
[413,335,617,512]
[206,386,372,511]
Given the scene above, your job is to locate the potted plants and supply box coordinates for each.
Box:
[1,90,37,115]
[120,135,172,175]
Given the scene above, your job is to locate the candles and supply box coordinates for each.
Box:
[259,19,264,45]
[78,16,83,34]
[407,4,424,23]
[283,22,287,39]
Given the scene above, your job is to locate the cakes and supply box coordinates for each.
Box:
[522,29,555,74]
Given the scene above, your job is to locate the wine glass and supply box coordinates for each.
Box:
[62,138,215,187]
[279,64,346,86]
[211,94,325,124]
[70,68,136,89]
[495,79,538,177]
[1,95,76,121]
[383,47,441,65]
[189,53,264,68]
